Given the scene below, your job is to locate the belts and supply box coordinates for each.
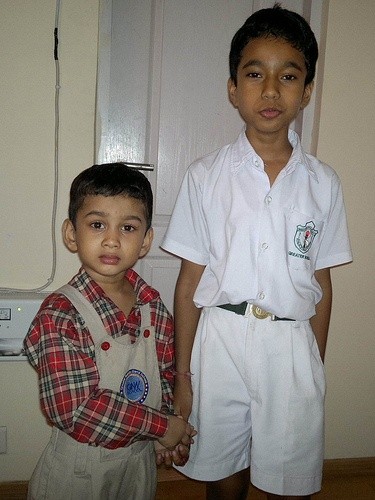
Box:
[216,301,295,321]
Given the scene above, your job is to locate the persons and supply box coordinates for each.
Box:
[151,3,353,500]
[27,163,197,500]
